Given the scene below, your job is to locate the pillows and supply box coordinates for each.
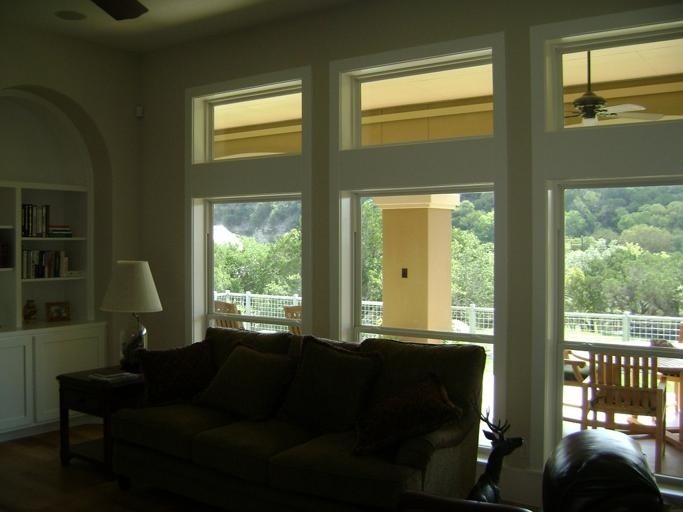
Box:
[195,344,302,423]
[118,338,221,408]
[354,364,464,452]
[274,335,382,433]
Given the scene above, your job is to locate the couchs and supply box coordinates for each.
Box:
[109,329,483,512]
[395,428,666,512]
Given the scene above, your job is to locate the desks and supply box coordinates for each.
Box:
[570,348,683,454]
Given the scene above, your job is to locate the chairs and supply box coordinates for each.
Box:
[282,305,306,336]
[561,348,597,432]
[580,346,667,475]
[215,300,243,329]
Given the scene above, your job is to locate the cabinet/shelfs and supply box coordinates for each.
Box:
[0,180,95,330]
[0,314,112,443]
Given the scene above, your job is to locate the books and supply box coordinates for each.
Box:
[88,368,137,383]
[21,248,81,278]
[22,204,73,238]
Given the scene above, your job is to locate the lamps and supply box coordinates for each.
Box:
[96,259,164,371]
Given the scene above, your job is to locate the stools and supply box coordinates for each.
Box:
[55,367,147,481]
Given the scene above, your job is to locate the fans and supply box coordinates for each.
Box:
[564,51,664,127]
